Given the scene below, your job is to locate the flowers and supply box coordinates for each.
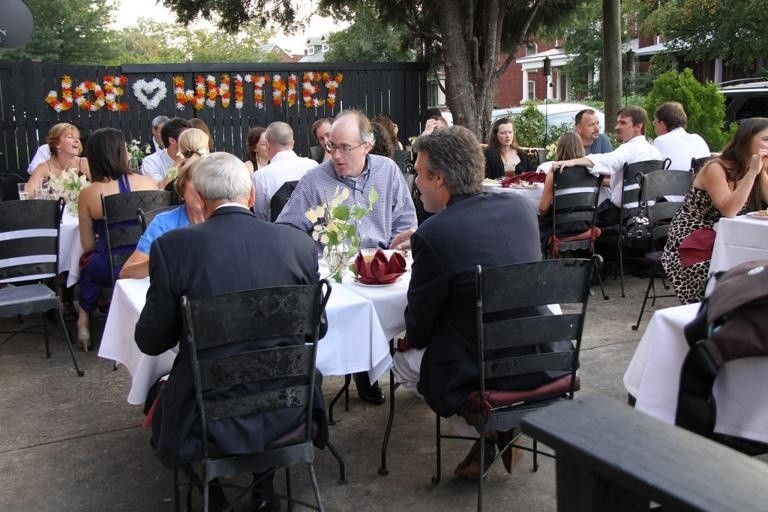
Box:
[45,71,344,112]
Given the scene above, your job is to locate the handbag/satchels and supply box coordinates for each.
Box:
[625,215,652,248]
[703,261,768,368]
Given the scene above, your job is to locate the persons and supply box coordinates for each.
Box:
[661,117,768,306]
[271,109,419,405]
[27,100,717,352]
[132,152,325,508]
[390,125,584,483]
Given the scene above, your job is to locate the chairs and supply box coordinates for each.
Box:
[164,278,346,511]
[0,144,726,381]
[384,252,604,512]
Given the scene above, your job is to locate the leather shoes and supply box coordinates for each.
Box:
[355,377,385,405]
[455,438,495,486]
[497,426,522,473]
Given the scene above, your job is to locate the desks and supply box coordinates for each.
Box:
[653,209,768,455]
[113,247,412,482]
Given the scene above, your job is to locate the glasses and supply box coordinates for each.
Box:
[326,140,365,154]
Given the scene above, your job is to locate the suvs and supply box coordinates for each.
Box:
[712,77,767,132]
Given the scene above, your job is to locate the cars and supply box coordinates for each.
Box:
[440,112,453,127]
[491,103,605,141]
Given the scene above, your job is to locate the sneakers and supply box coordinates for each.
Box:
[63,301,75,320]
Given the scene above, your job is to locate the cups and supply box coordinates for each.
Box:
[168,169,176,179]
[17,182,29,200]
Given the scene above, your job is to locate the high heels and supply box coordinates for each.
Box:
[76,327,92,352]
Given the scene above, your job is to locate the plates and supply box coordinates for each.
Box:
[746,209,768,221]
